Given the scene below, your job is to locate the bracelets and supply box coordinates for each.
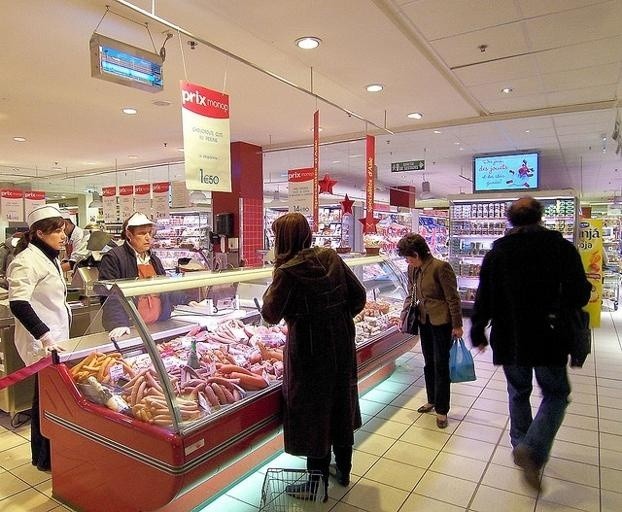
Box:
[40,332,52,342]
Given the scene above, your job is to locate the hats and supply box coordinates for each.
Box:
[27,204,72,231]
[11,237,22,248]
[128,212,154,226]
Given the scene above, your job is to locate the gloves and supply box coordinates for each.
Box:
[42,334,66,355]
[188,301,208,310]
[108,326,130,338]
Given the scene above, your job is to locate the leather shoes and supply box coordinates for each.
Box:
[417,405,448,428]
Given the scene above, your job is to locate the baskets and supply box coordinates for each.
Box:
[257,466,325,511]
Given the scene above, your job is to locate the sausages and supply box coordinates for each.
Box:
[68,351,268,426]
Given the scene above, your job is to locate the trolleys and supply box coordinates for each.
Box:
[254,464,330,512]
[600,266,622,312]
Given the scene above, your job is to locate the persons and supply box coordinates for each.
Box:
[601,246,609,305]
[77,223,119,267]
[0,231,24,289]
[58,210,86,272]
[471,196,595,491]
[397,233,464,428]
[7,201,74,473]
[97,212,199,339]
[261,213,368,499]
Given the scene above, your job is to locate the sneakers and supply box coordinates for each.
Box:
[31,458,51,472]
[285,463,351,504]
[512,442,545,494]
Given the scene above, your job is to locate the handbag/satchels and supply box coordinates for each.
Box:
[400,305,419,335]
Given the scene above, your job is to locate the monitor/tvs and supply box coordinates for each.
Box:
[472,150,539,194]
